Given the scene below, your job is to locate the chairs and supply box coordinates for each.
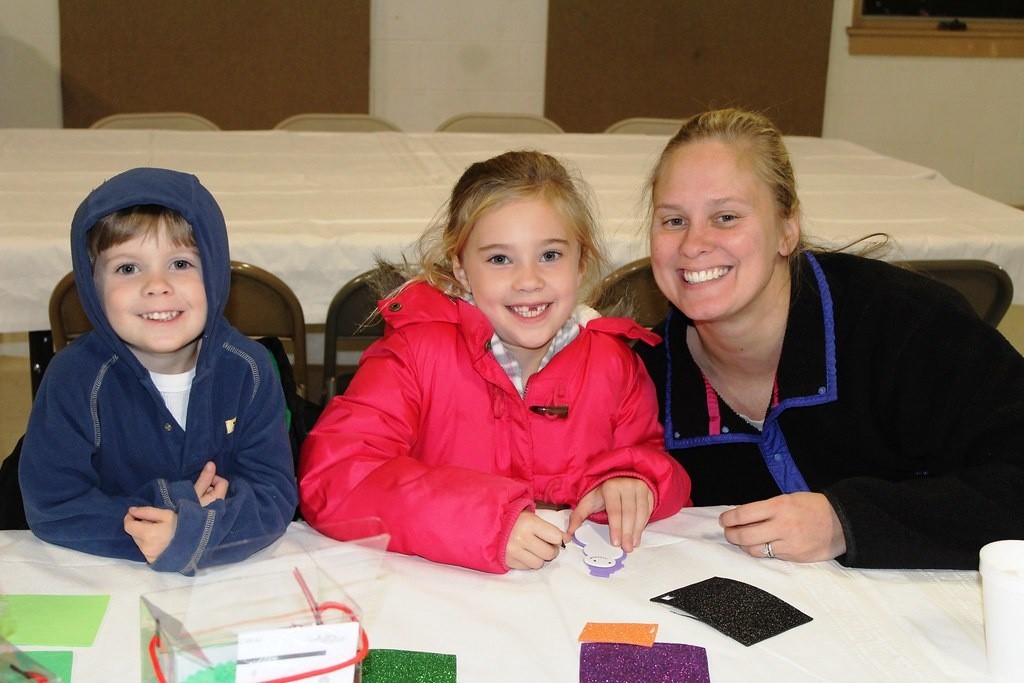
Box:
[89,112,220,131]
[435,112,563,134]
[319,263,443,412]
[582,259,675,350]
[272,113,402,133]
[603,118,690,135]
[890,258,1014,329]
[47,260,310,401]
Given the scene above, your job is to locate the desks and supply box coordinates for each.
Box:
[0,128,1024,466]
[0,505,1024,683]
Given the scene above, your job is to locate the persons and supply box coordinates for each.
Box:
[631,106,1024,571]
[298,150,690,575]
[19,167,298,577]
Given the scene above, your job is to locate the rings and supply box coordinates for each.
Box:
[763,543,774,558]
